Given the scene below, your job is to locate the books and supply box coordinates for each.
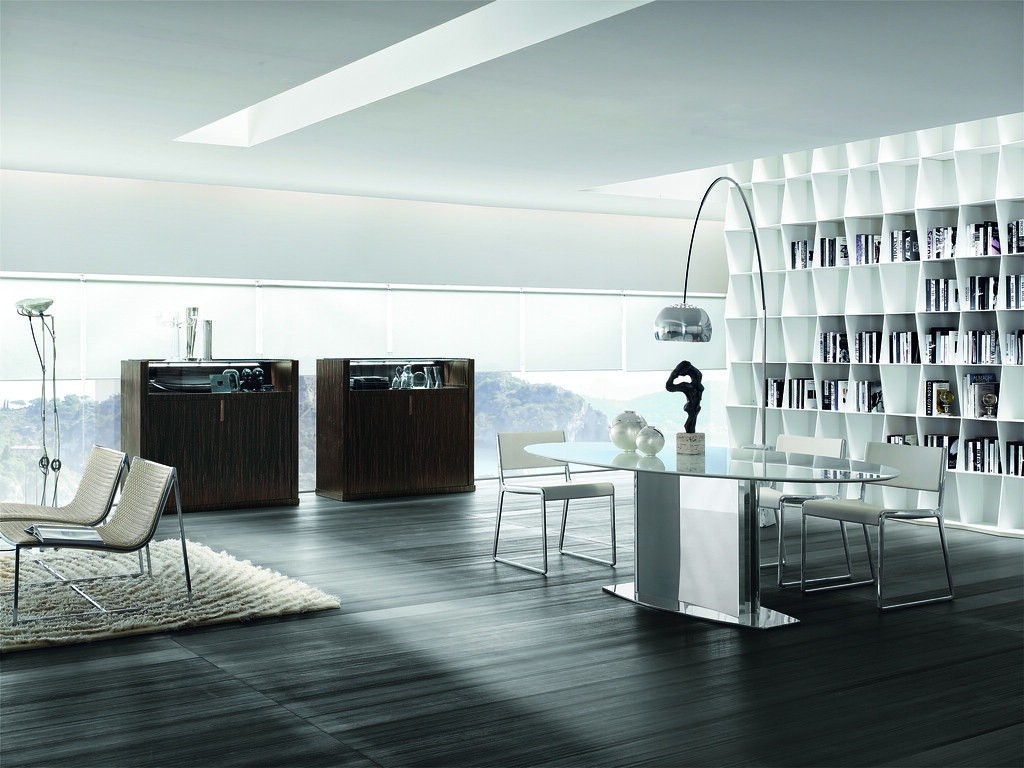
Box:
[758,216,1024,477]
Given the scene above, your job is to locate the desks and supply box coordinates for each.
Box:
[523,441,900,629]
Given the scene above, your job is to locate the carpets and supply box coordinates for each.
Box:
[0,538,341,654]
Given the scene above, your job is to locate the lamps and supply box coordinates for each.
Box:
[654,175,767,447]
[15,298,63,509]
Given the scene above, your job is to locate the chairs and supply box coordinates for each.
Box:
[756,433,956,611]
[0,444,194,627]
[491,430,616,575]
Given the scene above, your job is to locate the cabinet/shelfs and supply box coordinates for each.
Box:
[723,111,1024,538]
[120,358,300,515]
[316,358,475,502]
